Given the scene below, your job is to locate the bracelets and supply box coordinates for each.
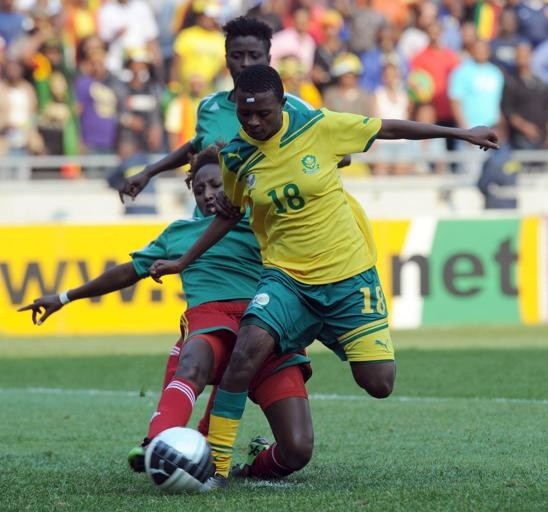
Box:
[60,291,70,305]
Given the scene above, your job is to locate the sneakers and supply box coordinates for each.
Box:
[229,434,270,479]
[197,472,226,495]
[127,436,149,473]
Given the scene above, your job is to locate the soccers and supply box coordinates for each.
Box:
[145,427,212,495]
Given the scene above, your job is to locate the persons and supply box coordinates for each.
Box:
[149,65,500,492]
[119,15,351,438]
[17,145,312,481]
